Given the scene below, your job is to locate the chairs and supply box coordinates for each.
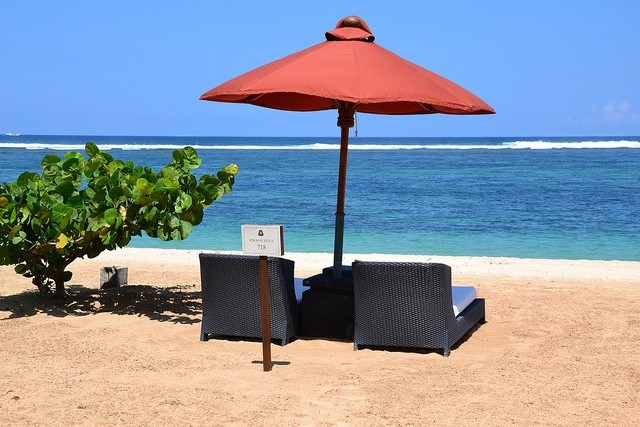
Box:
[351,261,487,359]
[196,251,305,348]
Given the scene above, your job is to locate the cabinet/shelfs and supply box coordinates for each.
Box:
[301,268,353,342]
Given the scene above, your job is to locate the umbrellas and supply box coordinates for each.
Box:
[199,15,496,268]
